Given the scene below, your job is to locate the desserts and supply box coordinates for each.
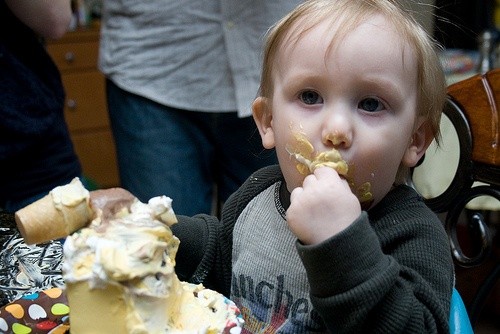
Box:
[60,195,186,334]
[15,176,99,246]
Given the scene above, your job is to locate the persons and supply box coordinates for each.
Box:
[88,0,465,334]
[0,0,88,222]
[100,1,310,225]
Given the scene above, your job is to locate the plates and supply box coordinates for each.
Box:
[0,282,243,334]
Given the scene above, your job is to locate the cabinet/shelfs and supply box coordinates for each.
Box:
[44,18,121,189]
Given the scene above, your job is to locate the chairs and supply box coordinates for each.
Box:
[409,92,500,334]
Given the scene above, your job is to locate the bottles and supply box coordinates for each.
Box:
[477,30,497,74]
